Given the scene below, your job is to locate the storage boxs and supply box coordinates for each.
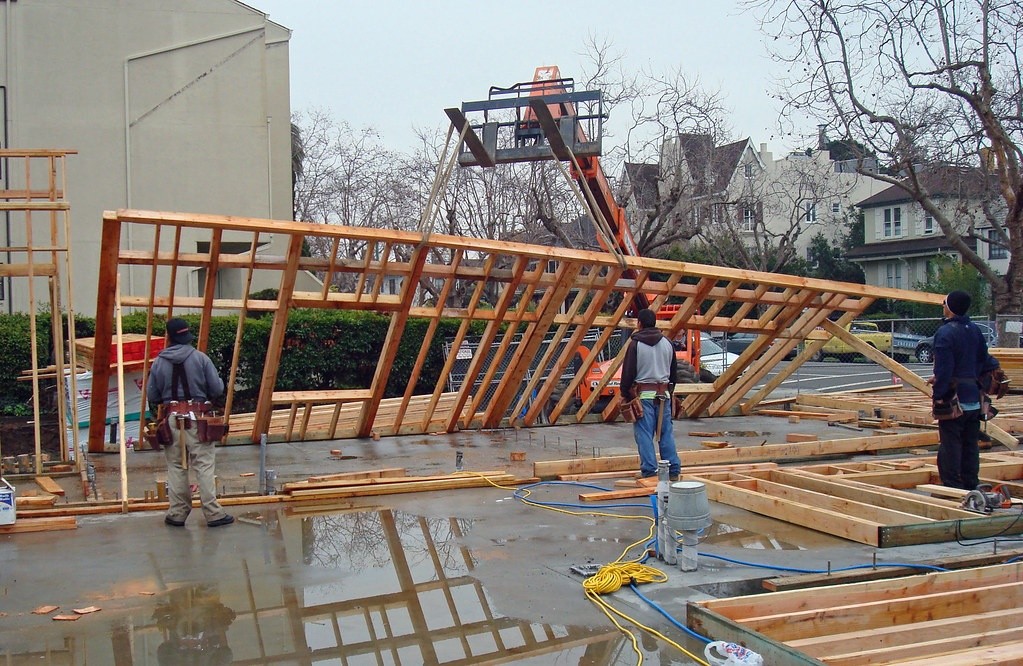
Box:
[0,477,16,526]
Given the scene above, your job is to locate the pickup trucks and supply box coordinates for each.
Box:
[804,320,927,365]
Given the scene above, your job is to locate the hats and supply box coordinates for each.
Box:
[945,291,971,317]
[166,317,194,344]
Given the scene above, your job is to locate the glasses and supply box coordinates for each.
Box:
[943,300,946,306]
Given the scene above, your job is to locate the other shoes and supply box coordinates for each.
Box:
[208,515,235,527]
[659,474,679,481]
[165,514,186,527]
[634,472,658,478]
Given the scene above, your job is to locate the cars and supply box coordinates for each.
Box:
[716,333,761,356]
[691,335,750,384]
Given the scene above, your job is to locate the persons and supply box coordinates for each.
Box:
[147,318,234,526]
[619,309,681,481]
[671,328,687,351]
[926,290,999,500]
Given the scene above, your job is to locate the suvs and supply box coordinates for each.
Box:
[915,320,1023,363]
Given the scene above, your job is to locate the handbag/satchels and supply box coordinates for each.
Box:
[931,389,963,419]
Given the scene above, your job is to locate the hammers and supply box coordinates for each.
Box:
[654,395,670,442]
[828,421,863,431]
[173,413,191,470]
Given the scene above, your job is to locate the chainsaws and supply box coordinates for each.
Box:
[962,483,1013,515]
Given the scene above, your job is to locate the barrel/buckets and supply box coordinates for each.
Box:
[667,480,713,546]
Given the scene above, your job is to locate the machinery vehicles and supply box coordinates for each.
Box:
[442,64,704,417]
